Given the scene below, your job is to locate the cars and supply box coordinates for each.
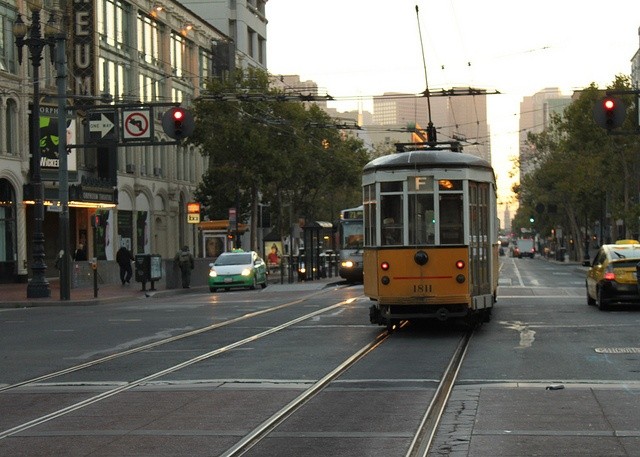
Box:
[582,240,640,310]
[208,249,268,292]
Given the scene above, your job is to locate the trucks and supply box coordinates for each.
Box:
[516,238,535,258]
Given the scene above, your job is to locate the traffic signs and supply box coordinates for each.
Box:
[83,109,118,142]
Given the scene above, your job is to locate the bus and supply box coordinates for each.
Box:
[338,205,364,285]
[361,140,500,333]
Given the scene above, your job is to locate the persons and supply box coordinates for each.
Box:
[175,245,194,288]
[116,239,136,284]
[267,243,280,264]
[206,237,225,257]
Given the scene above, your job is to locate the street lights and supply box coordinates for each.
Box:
[14,0,61,295]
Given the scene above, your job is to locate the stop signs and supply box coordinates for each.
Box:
[121,107,153,142]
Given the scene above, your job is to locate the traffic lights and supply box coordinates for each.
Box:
[173,106,182,142]
[605,92,616,131]
